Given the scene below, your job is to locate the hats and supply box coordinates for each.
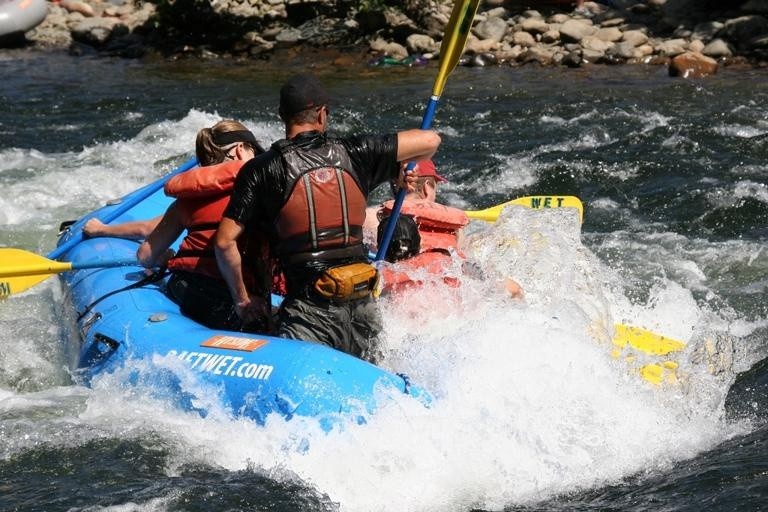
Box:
[279,73,331,120]
[378,214,420,251]
[419,159,446,182]
[213,131,265,155]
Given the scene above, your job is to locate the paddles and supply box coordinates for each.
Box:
[0,248,176,300]
[463,195,584,230]
[594,322,717,388]
[369,0,480,295]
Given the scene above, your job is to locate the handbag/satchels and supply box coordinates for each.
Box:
[314,262,378,303]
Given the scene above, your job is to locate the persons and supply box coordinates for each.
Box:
[214,72,442,362]
[364,157,525,319]
[84,217,288,297]
[136,120,285,336]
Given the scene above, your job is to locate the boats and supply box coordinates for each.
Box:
[46,170,437,447]
